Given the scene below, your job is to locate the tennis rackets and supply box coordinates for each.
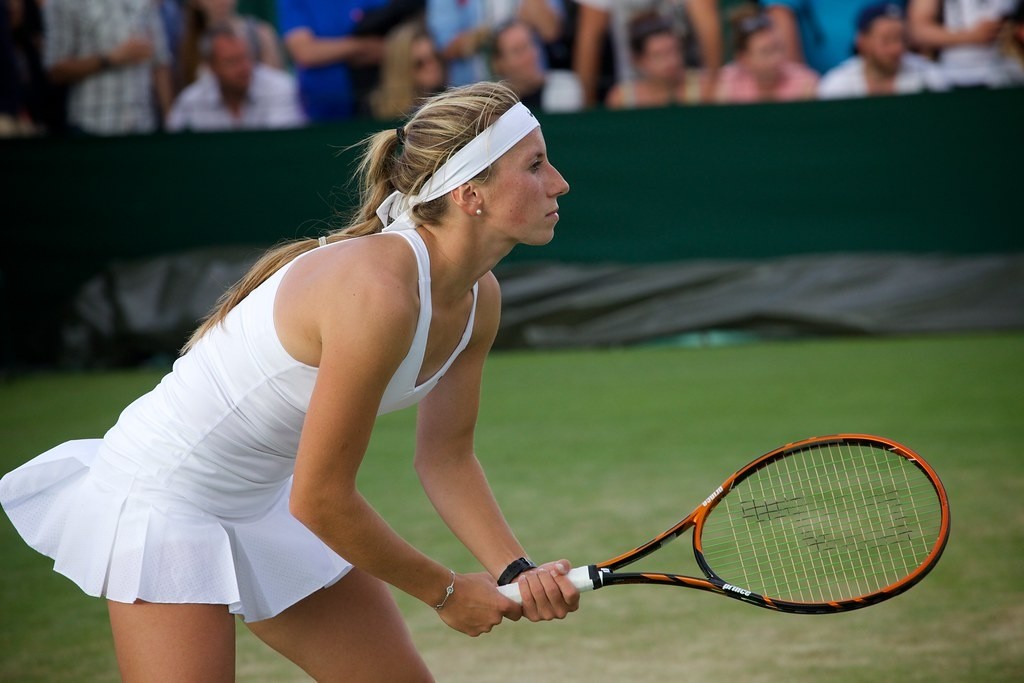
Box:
[495,435,951,614]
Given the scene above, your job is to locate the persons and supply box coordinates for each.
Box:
[0,79,581,683]
[0,0,1024,138]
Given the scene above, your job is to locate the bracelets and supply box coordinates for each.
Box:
[497,557,537,587]
[434,570,456,611]
